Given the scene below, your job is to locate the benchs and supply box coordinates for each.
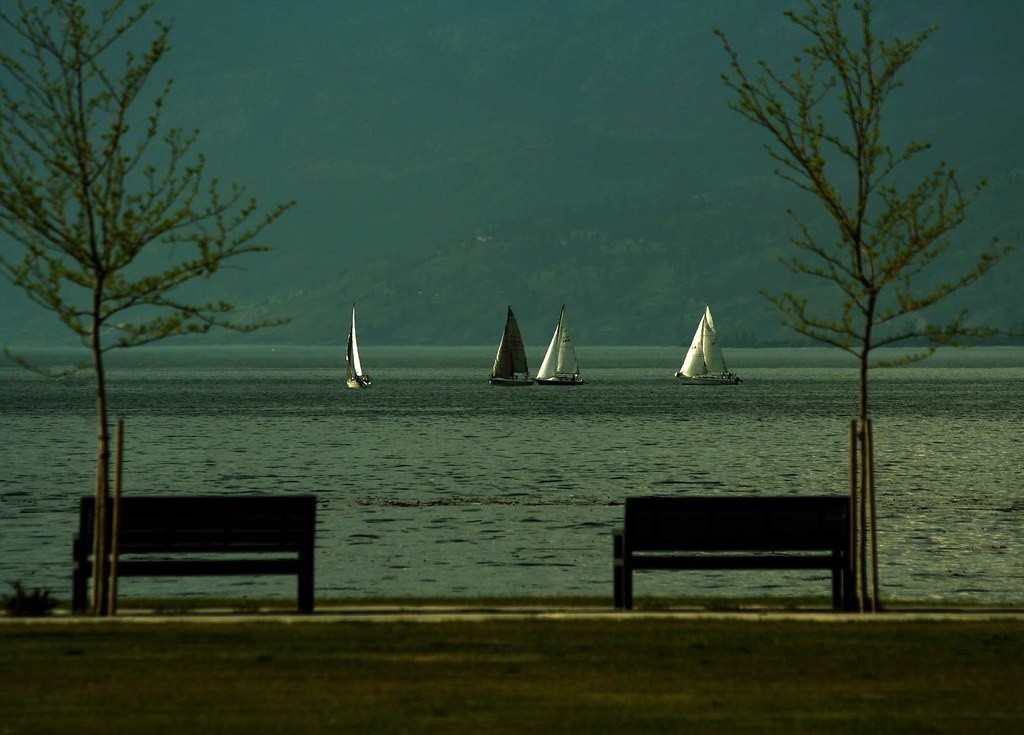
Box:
[70,496,318,616]
[611,496,854,611]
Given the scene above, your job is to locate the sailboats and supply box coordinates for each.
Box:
[675,304,745,386]
[345,303,371,389]
[535,304,587,385]
[488,304,534,386]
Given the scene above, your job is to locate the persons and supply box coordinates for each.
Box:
[561,372,579,381]
[361,373,371,384]
[720,368,735,382]
